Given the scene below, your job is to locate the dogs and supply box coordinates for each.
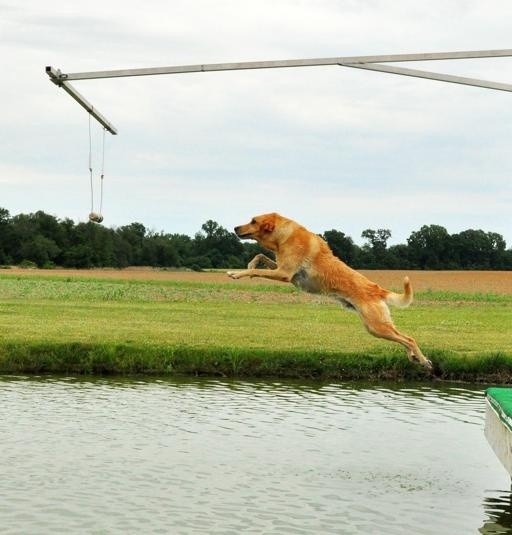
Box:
[226,212,433,372]
